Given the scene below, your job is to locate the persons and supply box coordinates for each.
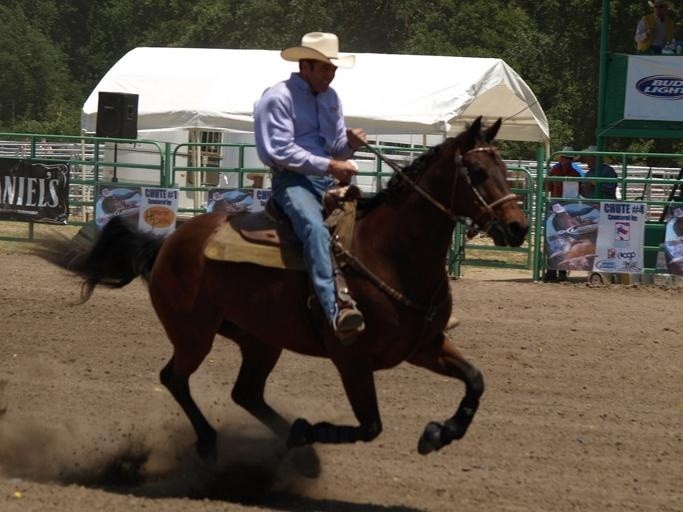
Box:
[19,137,54,155]
[665,205,682,274]
[547,204,600,251]
[253,32,370,347]
[582,146,616,199]
[634,0,675,54]
[99,186,140,225]
[542,148,580,282]
[248,174,263,189]
[209,190,253,214]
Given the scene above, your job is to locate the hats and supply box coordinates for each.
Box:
[280,32,355,69]
[581,146,597,152]
[554,146,581,161]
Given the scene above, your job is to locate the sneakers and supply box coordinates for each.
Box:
[333,308,362,346]
[444,316,459,329]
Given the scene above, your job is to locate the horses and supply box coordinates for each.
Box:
[21,115,531,479]
